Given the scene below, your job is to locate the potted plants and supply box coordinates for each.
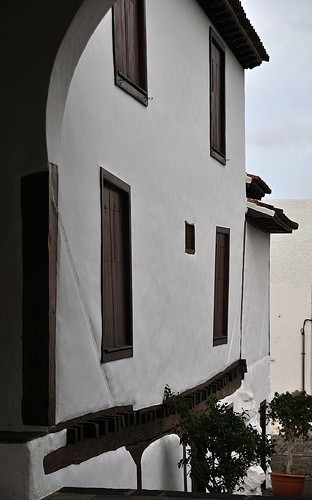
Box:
[257,390,312,500]
